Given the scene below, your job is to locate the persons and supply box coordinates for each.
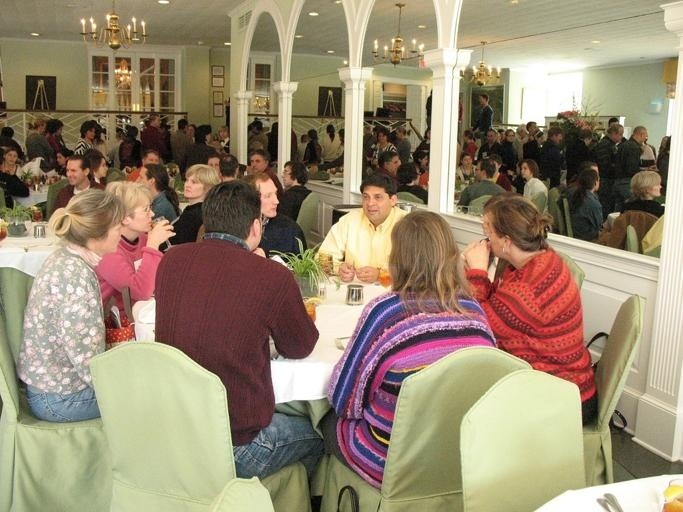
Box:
[462,195,599,430]
[15,188,128,424]
[151,179,324,485]
[2,112,408,285]
[407,91,672,251]
[325,210,498,489]
[247,95,432,203]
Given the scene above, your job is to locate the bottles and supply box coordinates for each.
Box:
[318,284,328,305]
[32,224,45,239]
[346,283,364,306]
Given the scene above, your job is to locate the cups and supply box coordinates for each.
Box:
[24,175,62,192]
[301,296,316,323]
[327,262,341,293]
[318,252,334,279]
[378,266,393,289]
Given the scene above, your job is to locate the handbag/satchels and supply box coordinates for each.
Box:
[105,287,136,349]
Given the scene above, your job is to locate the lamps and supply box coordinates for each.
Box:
[460,39,503,88]
[371,4,425,70]
[256,95,271,109]
[114,58,135,89]
[80,0,147,51]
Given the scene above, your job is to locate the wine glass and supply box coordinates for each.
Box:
[329,167,336,179]
[0,219,8,249]
[150,215,178,251]
[339,167,343,177]
[32,207,43,226]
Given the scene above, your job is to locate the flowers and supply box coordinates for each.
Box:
[557,110,600,132]
